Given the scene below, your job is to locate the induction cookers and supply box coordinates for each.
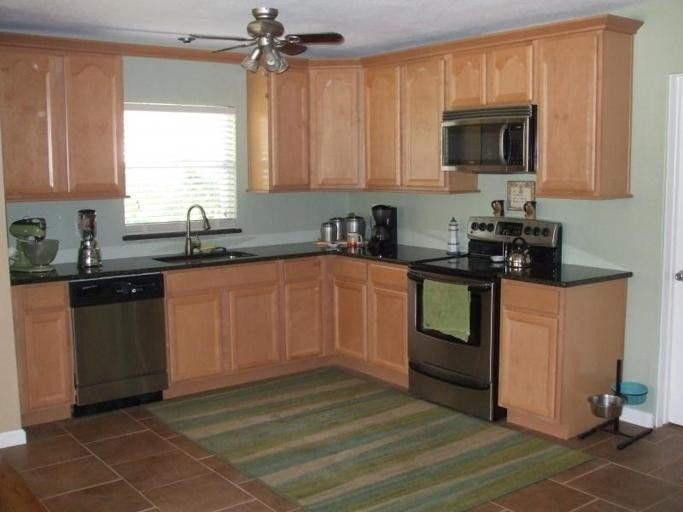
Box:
[406,253,538,287]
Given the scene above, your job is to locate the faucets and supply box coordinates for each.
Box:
[184,205,211,256]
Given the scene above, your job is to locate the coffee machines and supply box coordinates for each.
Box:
[367,203,398,251]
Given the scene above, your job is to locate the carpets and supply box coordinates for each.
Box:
[142,364,594,511]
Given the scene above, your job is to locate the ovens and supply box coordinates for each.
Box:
[405,268,508,424]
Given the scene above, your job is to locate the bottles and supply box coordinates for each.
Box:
[320,215,367,242]
[447,216,459,255]
[448,257,460,268]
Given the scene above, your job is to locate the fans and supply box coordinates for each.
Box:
[177,7,345,77]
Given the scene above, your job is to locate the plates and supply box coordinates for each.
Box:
[491,255,506,263]
[490,263,505,268]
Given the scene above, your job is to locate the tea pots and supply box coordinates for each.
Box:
[508,237,533,269]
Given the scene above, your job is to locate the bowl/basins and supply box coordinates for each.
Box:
[587,394,626,419]
[611,381,648,405]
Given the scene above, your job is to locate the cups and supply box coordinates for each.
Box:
[491,200,505,216]
[524,200,537,220]
[347,231,364,249]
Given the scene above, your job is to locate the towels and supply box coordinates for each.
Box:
[419,276,472,343]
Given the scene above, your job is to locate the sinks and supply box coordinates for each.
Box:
[152,250,259,263]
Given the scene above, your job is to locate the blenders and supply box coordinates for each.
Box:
[6,217,60,274]
[76,208,103,270]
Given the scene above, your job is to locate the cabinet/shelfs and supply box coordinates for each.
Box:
[536,28,635,201]
[442,39,535,113]
[0,33,130,202]
[366,48,485,192]
[13,281,76,428]
[163,258,281,403]
[247,66,310,196]
[279,253,327,378]
[309,66,365,193]
[325,257,408,387]
[499,278,630,441]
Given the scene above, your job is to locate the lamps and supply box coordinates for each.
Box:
[240,45,288,74]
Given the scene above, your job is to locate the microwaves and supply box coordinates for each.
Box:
[439,107,537,177]
